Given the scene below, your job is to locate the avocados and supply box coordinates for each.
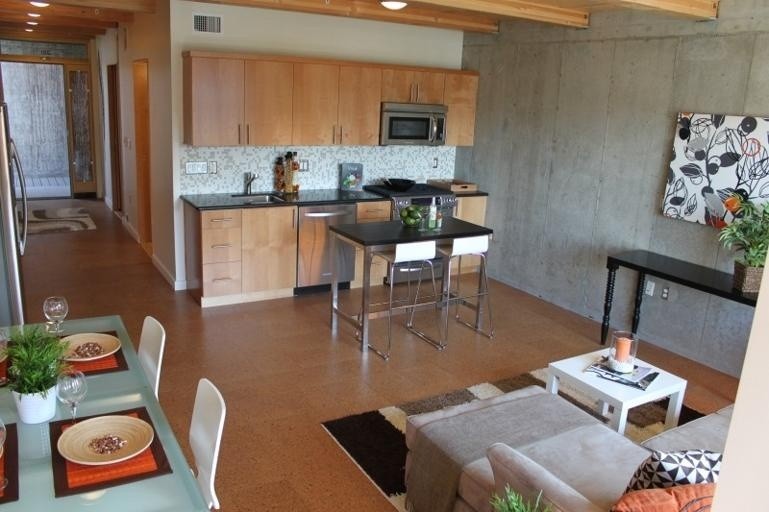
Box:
[415,218,422,225]
[405,217,415,225]
[406,206,415,212]
[409,211,418,218]
[400,208,409,218]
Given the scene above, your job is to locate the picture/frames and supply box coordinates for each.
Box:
[660,111,769,230]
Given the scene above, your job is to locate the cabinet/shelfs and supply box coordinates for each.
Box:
[351,202,390,289]
[452,198,488,274]
[293,61,381,146]
[241,206,297,293]
[183,57,293,148]
[183,201,241,296]
[298,205,357,287]
[447,74,479,147]
[381,68,445,107]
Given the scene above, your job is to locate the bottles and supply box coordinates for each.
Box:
[274,151,299,193]
[427,196,437,230]
[436,213,442,228]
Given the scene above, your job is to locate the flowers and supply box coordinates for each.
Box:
[719,191,769,267]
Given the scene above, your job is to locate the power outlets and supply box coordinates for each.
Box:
[645,280,656,296]
[185,162,207,175]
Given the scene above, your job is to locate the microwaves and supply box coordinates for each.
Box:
[380,103,448,147]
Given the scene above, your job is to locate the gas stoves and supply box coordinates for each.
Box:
[364,184,454,198]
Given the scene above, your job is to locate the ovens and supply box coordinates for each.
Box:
[393,196,458,267]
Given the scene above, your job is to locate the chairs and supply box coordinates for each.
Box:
[136,315,165,399]
[189,377,226,510]
[410,234,495,345]
[355,240,443,358]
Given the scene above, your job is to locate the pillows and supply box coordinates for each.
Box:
[624,448,724,489]
[608,484,718,512]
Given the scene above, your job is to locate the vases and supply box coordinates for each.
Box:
[733,259,765,294]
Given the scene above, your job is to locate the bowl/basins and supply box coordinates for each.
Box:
[384,179,416,193]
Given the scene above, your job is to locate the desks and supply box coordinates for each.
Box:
[601,249,760,346]
[0,315,209,512]
[327,217,495,353]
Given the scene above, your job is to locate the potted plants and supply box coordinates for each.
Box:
[0,323,69,425]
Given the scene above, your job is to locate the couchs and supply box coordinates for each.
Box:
[405,385,735,512]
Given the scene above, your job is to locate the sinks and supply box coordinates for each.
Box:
[231,193,284,205]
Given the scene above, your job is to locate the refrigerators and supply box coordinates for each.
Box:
[1,103,28,327]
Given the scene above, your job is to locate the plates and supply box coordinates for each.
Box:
[56,333,121,361]
[56,415,155,464]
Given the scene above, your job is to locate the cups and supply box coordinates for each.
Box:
[42,297,68,333]
[0,420,8,492]
[57,371,89,420]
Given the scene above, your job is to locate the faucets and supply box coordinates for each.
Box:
[243,172,259,196]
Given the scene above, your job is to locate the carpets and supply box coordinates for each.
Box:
[17,205,96,235]
[320,366,707,512]
